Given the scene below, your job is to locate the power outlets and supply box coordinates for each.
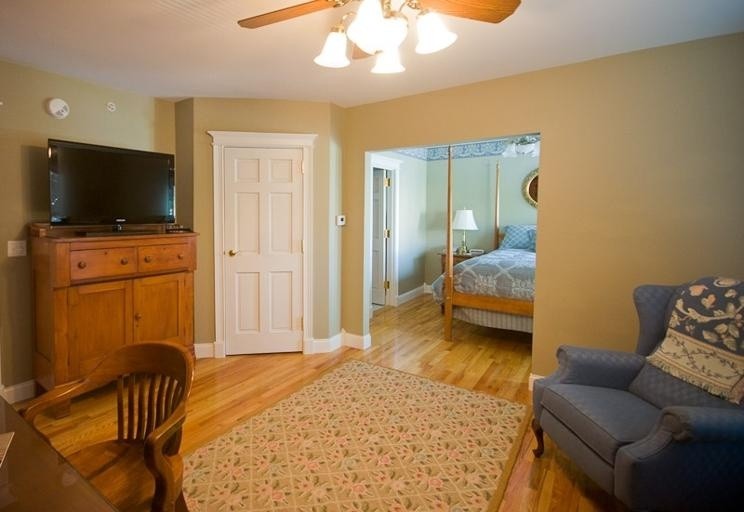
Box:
[7,239,27,258]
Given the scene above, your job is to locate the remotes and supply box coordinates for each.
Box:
[166,228,191,233]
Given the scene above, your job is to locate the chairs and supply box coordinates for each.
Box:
[25,341,196,512]
[531,282,744,512]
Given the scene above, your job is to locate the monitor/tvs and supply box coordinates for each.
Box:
[46,138,175,237]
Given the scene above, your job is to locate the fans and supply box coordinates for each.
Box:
[238,0,522,60]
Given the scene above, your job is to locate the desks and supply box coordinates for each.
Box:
[0,395,119,512]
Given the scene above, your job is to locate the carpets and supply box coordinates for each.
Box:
[183,356,532,510]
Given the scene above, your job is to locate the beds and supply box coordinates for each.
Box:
[431,142,539,342]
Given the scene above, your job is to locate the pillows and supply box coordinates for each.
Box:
[497,223,536,249]
[528,229,535,252]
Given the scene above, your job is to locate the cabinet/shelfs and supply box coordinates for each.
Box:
[29,232,204,418]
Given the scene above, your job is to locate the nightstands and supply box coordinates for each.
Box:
[436,251,473,274]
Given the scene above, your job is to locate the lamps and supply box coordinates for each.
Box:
[313,1,459,74]
[452,206,480,253]
[502,135,540,160]
